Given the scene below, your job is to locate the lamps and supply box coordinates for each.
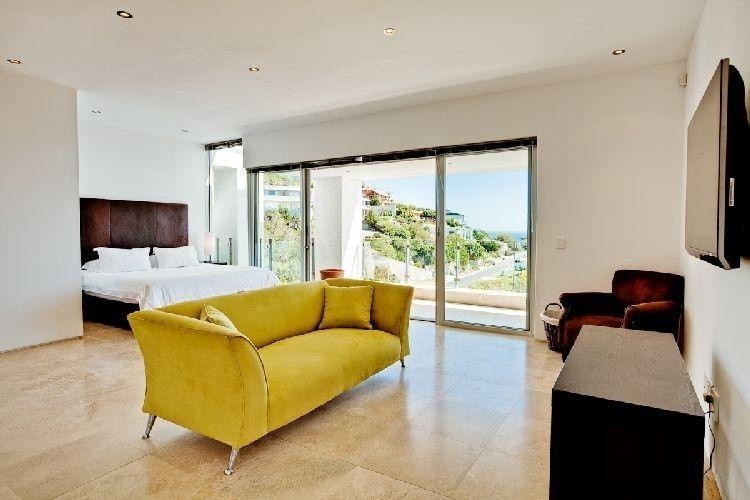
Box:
[203,232,215,263]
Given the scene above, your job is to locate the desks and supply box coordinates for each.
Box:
[549,325,705,500]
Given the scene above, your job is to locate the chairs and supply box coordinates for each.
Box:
[558,270,685,362]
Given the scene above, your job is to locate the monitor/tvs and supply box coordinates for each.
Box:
[684,57,750,270]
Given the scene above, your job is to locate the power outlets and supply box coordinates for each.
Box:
[709,388,719,423]
[702,372,714,418]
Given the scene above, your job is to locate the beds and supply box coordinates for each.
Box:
[80,197,280,331]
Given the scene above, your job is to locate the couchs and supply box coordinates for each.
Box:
[128,277,414,476]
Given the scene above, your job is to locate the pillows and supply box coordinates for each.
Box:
[93,247,152,272]
[200,303,239,332]
[318,284,375,330]
[152,246,199,269]
